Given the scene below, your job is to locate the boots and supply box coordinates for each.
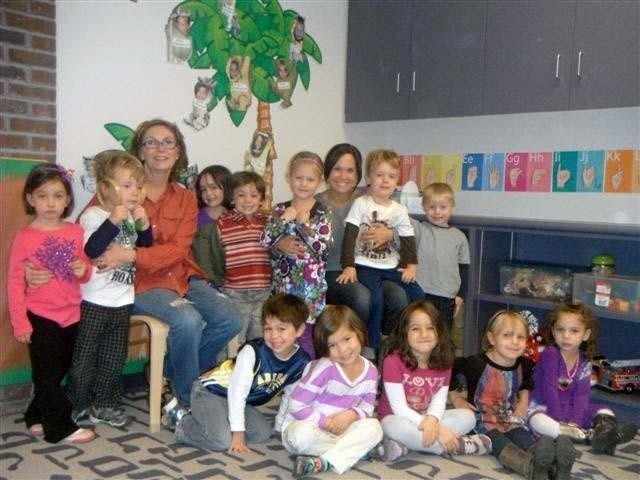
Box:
[527,435,576,476]
[586,412,619,456]
[617,418,636,448]
[499,434,556,479]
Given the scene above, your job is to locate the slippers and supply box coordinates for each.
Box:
[27,424,44,436]
[63,426,96,444]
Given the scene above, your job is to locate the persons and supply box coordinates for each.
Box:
[336,148,425,372]
[76,120,244,432]
[259,151,334,362]
[280,306,384,480]
[6,163,94,444]
[449,311,577,480]
[369,300,493,462]
[195,165,232,229]
[160,293,311,454]
[214,171,273,366]
[67,149,149,430]
[417,183,471,365]
[313,143,420,346]
[527,300,638,456]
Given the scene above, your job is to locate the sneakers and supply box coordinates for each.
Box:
[457,433,493,455]
[384,438,408,462]
[361,443,385,461]
[89,405,132,427]
[160,396,189,431]
[71,407,89,424]
[291,455,330,478]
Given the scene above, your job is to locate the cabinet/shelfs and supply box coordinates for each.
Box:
[409,212,639,421]
[485,0,639,114]
[344,1,486,124]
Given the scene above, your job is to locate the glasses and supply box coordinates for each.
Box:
[138,137,179,149]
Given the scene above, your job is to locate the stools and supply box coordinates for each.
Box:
[129,313,172,434]
